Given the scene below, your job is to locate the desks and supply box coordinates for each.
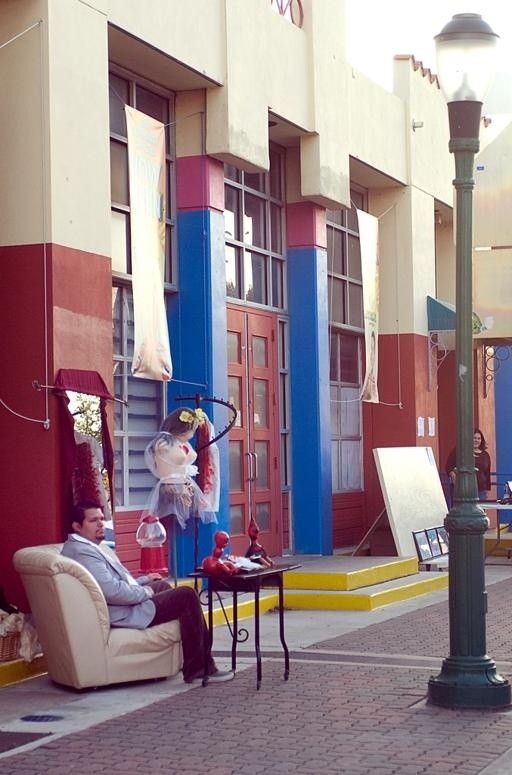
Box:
[479,502,512,561]
[187,563,305,694]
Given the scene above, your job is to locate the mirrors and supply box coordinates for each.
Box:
[63,389,116,551]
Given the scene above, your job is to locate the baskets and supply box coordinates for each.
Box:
[0,630,22,662]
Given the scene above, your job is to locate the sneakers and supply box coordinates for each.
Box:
[191,669,235,684]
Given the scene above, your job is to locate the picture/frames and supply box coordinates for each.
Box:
[412,525,450,562]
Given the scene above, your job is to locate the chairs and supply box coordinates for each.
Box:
[14,541,186,692]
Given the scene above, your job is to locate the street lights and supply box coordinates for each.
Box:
[427,9,512,714]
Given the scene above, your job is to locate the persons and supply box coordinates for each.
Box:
[445,426,494,510]
[138,405,221,527]
[59,500,235,683]
